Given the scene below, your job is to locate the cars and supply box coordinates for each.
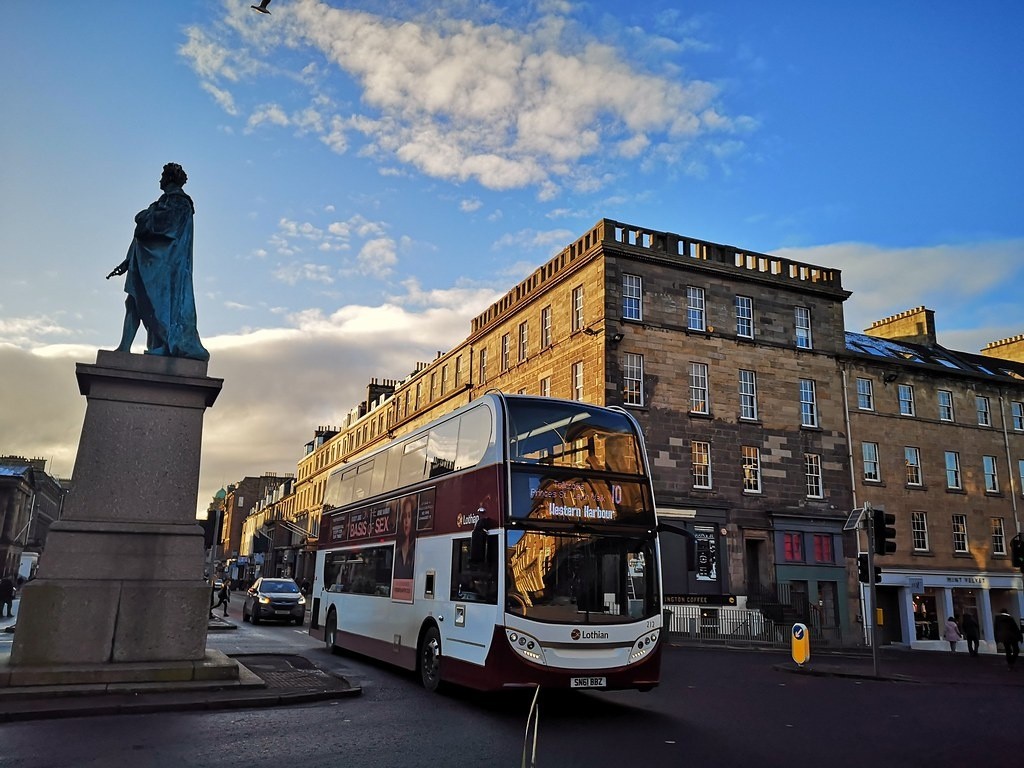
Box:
[214,578,224,588]
[243,576,307,627]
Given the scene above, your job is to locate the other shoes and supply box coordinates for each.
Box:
[7,614,14,617]
[0,614,3,618]
[209,616,215,619]
[224,614,229,617]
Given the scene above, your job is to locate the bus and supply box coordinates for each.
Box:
[308,388,699,696]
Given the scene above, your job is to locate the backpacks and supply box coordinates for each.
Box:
[218,589,223,598]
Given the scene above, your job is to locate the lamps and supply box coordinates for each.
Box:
[610,333,625,343]
[465,384,475,389]
[884,371,898,383]
[583,328,596,336]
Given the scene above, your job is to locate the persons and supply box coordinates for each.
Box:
[209,574,231,619]
[962,612,980,657]
[0,573,15,619]
[944,617,962,652]
[994,608,1023,665]
[105,163,210,360]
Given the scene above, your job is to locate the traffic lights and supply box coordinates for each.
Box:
[874,508,897,556]
[856,553,883,584]
